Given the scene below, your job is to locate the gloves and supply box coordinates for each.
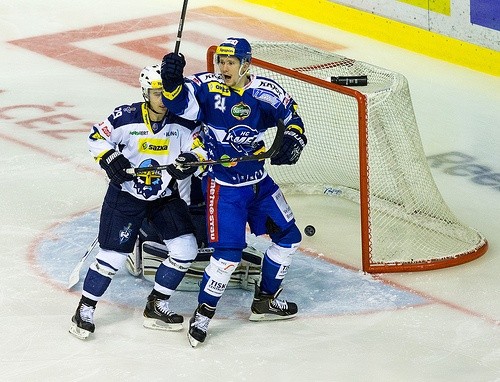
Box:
[161,53,186,88]
[270,128,307,165]
[166,152,200,180]
[100,149,133,183]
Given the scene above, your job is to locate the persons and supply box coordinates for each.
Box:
[67,65,265,340]
[160,38,307,348]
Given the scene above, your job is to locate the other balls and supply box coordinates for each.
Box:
[305,225,316,237]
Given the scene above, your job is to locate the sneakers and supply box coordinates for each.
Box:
[187,303,217,347]
[143,293,184,330]
[249,281,298,321]
[69,299,96,341]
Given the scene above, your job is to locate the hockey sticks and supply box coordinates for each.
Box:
[67,234,98,290]
[124,118,286,173]
[175,1,188,54]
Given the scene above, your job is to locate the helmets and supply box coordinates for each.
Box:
[139,64,162,102]
[213,38,251,65]
